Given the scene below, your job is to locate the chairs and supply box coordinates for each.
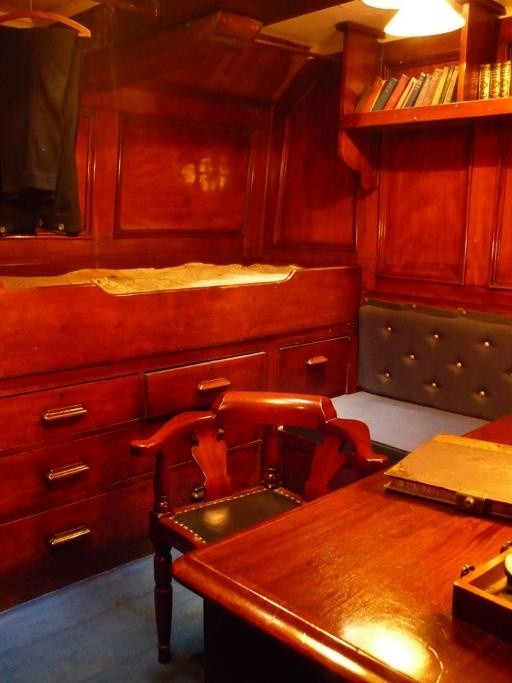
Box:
[129,390,387,661]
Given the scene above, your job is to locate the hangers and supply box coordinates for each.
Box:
[0,2,92,39]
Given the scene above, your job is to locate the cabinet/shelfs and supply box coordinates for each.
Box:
[335,17,512,191]
[1,319,358,613]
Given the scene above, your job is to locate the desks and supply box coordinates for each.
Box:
[169,411,512,682]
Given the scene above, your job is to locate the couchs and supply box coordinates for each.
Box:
[329,302,512,457]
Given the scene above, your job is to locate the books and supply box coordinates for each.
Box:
[381,432,511,520]
[478,63,490,101]
[490,62,502,99]
[470,67,478,99]
[502,60,512,99]
[354,65,459,113]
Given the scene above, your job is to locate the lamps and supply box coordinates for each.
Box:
[362,0,466,38]
